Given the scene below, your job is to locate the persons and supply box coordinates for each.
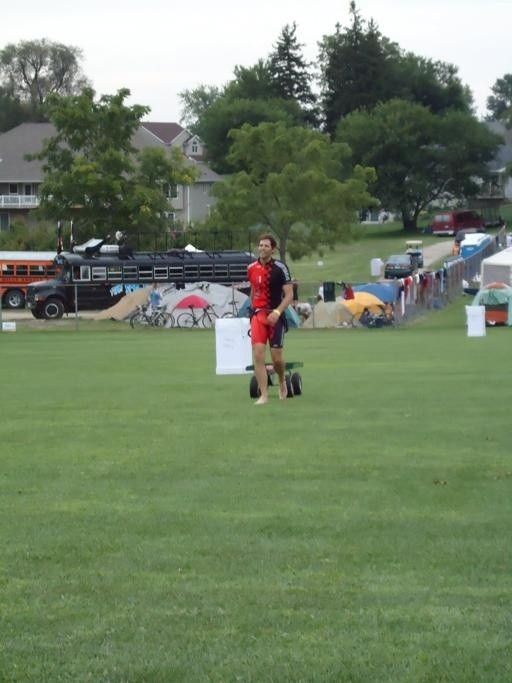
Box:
[451,240,461,258]
[244,235,293,404]
[114,229,134,260]
[405,244,414,253]
[414,245,421,254]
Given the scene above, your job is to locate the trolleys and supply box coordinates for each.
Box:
[246,325,303,399]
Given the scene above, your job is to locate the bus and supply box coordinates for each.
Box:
[0,221,258,320]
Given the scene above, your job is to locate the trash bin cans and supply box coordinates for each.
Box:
[481,282,508,325]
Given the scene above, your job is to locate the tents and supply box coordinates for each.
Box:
[90,280,300,328]
[301,283,401,328]
[470,279,512,327]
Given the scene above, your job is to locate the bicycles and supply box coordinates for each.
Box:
[130,299,239,329]
[351,303,395,329]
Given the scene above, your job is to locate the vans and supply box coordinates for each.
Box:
[431,210,485,235]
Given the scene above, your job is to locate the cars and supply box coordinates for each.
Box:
[454,228,491,256]
[485,216,503,227]
[385,240,423,279]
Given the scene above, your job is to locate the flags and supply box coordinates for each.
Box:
[56,220,64,253]
[69,221,78,254]
